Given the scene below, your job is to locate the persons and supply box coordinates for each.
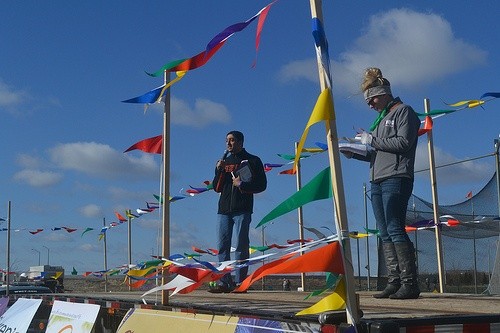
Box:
[335,67,421,301]
[208,130,267,295]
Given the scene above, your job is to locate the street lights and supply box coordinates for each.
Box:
[261,222,274,291]
[31,248,40,266]
[42,245,49,266]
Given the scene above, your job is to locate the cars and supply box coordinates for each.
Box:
[0,279,57,296]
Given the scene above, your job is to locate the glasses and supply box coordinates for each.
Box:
[366,96,376,107]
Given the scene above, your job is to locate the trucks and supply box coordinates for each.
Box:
[29,265,65,293]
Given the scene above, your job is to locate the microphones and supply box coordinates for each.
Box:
[222,149,229,161]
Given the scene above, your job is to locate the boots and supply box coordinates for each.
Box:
[373,241,401,298]
[389,241,421,299]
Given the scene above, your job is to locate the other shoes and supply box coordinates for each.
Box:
[230,287,246,293]
[207,284,231,293]
